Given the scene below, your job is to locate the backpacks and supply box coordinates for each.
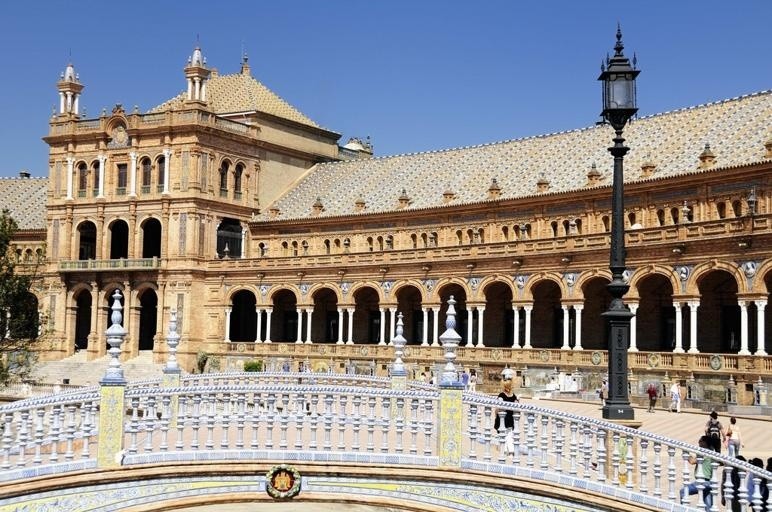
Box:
[707,420,720,440]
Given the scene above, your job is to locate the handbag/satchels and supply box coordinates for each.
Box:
[672,394,678,401]
[727,430,732,436]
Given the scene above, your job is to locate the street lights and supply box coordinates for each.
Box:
[597,19,642,429]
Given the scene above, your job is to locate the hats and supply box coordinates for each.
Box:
[710,412,717,419]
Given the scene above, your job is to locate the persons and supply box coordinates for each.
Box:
[726,415,744,457]
[420,367,434,385]
[631,220,642,229]
[493,380,519,450]
[647,382,658,413]
[679,434,714,512]
[722,455,772,511]
[461,369,477,392]
[601,381,608,406]
[502,364,514,380]
[605,380,609,392]
[705,411,726,453]
[283,362,289,372]
[669,381,683,413]
[299,365,302,372]
